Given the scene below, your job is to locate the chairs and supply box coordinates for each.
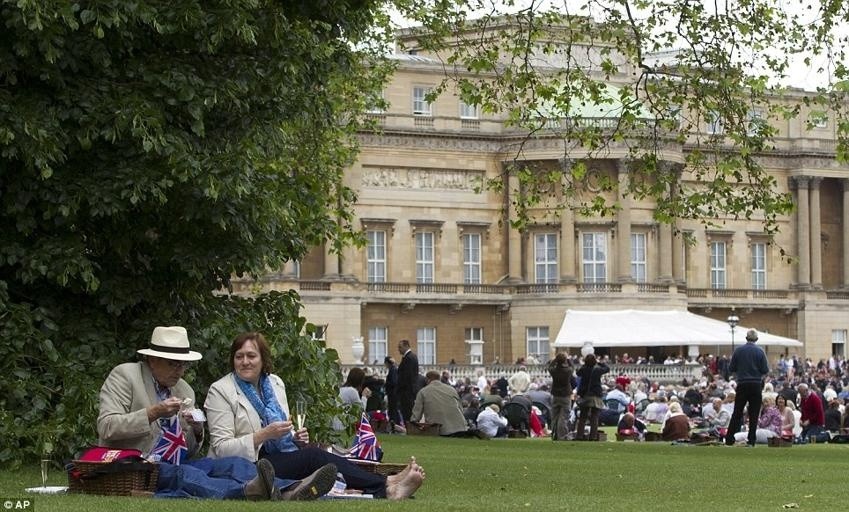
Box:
[465,398,652,443]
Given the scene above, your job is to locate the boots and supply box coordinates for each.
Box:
[280,461,339,504]
[241,455,283,502]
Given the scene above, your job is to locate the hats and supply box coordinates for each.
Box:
[135,325,204,362]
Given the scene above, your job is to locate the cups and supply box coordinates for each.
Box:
[810,435,817,444]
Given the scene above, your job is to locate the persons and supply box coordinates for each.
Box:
[725,327,770,446]
[97,324,338,500]
[205,330,425,502]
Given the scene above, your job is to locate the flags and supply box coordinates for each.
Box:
[348,409,384,464]
[148,415,189,467]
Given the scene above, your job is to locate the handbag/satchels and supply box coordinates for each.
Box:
[689,431,717,444]
[404,421,443,437]
[831,433,849,444]
[718,427,730,437]
[575,395,589,406]
[507,428,529,439]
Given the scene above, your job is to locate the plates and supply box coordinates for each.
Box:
[327,494,373,501]
[25,485,69,494]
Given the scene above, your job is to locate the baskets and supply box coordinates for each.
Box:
[347,457,408,478]
[63,460,161,497]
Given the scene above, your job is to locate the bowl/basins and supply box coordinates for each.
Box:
[767,437,792,447]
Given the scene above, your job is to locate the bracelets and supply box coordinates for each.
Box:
[194,427,204,437]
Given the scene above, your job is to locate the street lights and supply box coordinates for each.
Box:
[726,310,738,352]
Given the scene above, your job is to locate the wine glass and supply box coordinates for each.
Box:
[295,400,308,443]
[41,453,52,488]
